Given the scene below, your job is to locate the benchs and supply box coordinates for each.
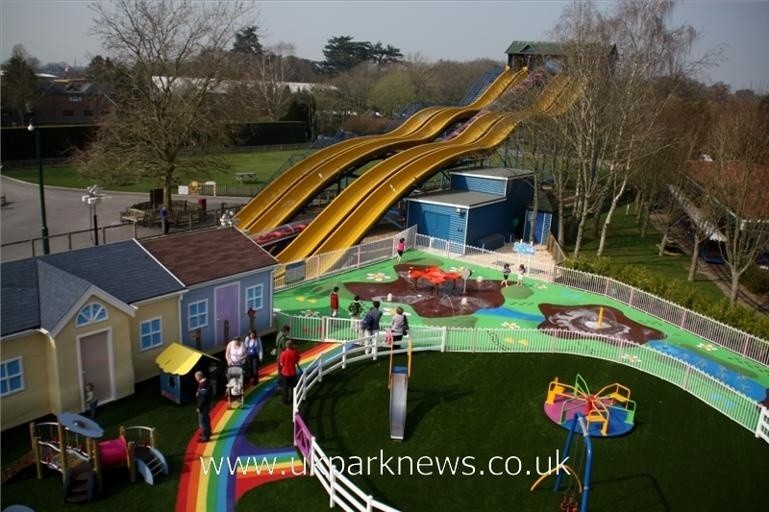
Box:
[120,208,146,226]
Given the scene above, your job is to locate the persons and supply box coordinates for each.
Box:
[85,383,98,419]
[501,264,511,287]
[396,238,406,265]
[225,325,301,404]
[195,371,212,442]
[160,207,169,219]
[331,286,339,328]
[390,307,407,359]
[517,264,526,287]
[348,295,363,333]
[365,301,382,360]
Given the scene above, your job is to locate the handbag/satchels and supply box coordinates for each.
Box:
[403,316,409,335]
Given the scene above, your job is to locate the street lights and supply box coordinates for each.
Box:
[28,111,52,255]
[80,184,112,248]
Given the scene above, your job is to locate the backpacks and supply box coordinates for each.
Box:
[361,313,375,330]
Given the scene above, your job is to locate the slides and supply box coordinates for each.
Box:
[390,373,408,440]
[229,64,588,291]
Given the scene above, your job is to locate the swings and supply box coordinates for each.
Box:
[560,433,587,512]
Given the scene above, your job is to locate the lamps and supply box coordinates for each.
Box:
[457,208,462,217]
